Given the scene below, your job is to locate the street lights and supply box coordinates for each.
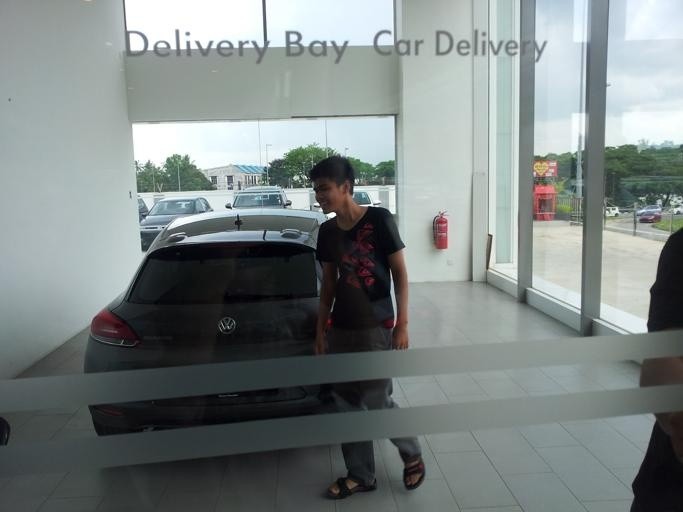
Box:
[151,162,157,192]
[177,159,184,192]
[310,142,320,169]
[264,143,272,186]
[344,146,350,160]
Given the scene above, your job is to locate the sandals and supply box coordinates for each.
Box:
[403,455,425,490]
[326,471,377,499]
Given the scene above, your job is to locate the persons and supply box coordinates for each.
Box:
[307,156,426,498]
[628,227,682,512]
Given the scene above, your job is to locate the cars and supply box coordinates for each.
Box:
[133,184,382,252]
[82,209,344,452]
[602,202,683,223]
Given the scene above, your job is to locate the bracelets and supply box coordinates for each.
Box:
[396,321,407,325]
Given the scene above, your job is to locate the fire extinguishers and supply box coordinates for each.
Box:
[433,209,449,249]
